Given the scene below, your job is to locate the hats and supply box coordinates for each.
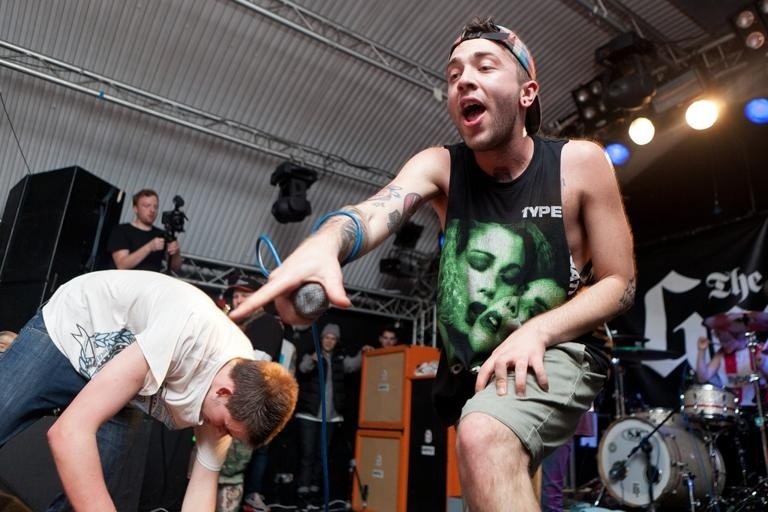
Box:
[320,323,340,338]
[223,277,263,304]
[450,24,542,135]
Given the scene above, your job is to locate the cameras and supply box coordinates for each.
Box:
[161,196,186,234]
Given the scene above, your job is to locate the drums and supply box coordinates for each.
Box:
[630,407,688,430]
[596,415,726,512]
[681,383,744,431]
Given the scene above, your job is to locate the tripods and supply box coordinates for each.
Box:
[579,370,625,506]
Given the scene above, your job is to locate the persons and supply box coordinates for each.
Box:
[0,268,303,511]
[342,325,400,377]
[219,277,284,510]
[436,219,538,338]
[271,311,298,377]
[467,274,566,356]
[292,321,363,501]
[227,15,640,511]
[106,188,181,273]
[696,313,768,510]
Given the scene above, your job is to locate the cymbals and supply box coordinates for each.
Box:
[612,346,672,361]
[701,312,768,342]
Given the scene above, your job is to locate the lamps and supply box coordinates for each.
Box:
[728,0,768,58]
[270,160,318,224]
[568,38,654,125]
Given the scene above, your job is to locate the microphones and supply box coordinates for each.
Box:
[293,282,330,321]
